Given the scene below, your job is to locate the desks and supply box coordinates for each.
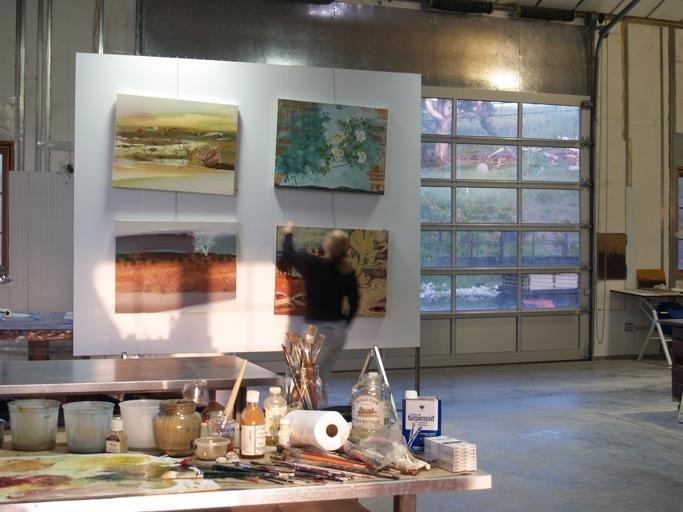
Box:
[609,284,682,369]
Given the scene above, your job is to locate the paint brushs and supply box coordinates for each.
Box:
[219,359,247,438]
[282,325,325,410]
[155,445,401,485]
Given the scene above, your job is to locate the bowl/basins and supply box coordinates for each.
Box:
[193,436,231,461]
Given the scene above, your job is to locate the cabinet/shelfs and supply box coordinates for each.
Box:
[656,319,682,412]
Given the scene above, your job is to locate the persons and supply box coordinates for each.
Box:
[278,220,361,408]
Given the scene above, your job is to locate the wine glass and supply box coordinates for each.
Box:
[181,378,210,418]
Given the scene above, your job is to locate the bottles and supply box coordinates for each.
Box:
[401,389,441,454]
[351,373,392,456]
[263,386,288,446]
[104,418,127,453]
[239,389,267,460]
[207,410,237,453]
[152,399,202,457]
[284,365,324,412]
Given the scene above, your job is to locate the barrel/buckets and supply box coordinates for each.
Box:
[119,400,163,449]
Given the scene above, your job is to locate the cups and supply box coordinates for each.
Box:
[0,418,6,446]
[117,398,166,451]
